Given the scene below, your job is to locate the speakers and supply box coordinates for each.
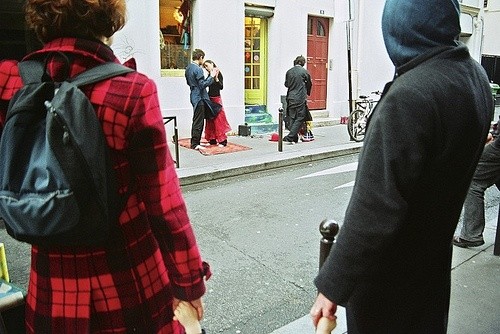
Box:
[239,125,251,136]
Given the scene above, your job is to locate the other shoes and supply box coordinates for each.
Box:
[218,143,223,147]
[206,143,210,147]
[195,145,206,149]
[453,233,484,247]
[200,142,205,146]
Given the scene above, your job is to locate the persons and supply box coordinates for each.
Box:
[184,48,219,151]
[282,55,313,145]
[204,59,232,148]
[452,112,500,250]
[309,0,496,334]
[0,1,212,334]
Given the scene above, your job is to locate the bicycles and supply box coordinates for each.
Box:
[347,90,382,142]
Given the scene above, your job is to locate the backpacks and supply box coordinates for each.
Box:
[0,52,135,246]
